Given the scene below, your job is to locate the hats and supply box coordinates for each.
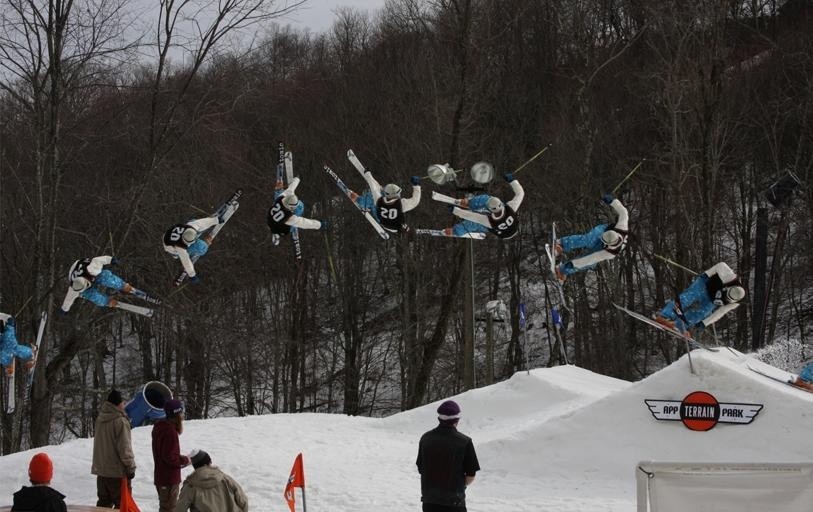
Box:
[30,453,52,481]
[438,401,460,415]
[108,391,122,406]
[164,400,182,415]
[190,448,210,468]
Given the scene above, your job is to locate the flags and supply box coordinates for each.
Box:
[283,452,303,511]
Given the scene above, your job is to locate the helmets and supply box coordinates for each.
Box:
[72,277,87,292]
[727,287,745,302]
[285,194,299,208]
[488,197,503,213]
[601,230,619,246]
[384,184,402,198]
[182,228,197,245]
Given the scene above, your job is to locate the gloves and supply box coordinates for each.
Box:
[218,216,224,223]
[696,321,704,332]
[604,193,614,204]
[60,308,69,316]
[320,221,328,230]
[409,176,420,185]
[506,174,513,182]
[111,257,117,263]
[701,273,710,282]
[8,318,15,326]
[192,276,199,283]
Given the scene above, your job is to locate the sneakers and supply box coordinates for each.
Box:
[445,227,452,235]
[6,347,34,377]
[109,283,131,308]
[351,191,358,202]
[657,315,691,340]
[204,234,212,244]
[460,197,469,206]
[796,376,813,391]
[554,239,566,280]
[274,180,283,189]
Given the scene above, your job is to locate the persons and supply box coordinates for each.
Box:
[550,190,629,283]
[0,313,35,371]
[172,448,249,511]
[150,398,196,511]
[264,174,329,239]
[799,359,812,387]
[57,252,133,318]
[651,259,746,342]
[9,452,68,511]
[347,165,423,238]
[89,388,137,509]
[161,212,224,286]
[415,399,480,511]
[439,173,526,244]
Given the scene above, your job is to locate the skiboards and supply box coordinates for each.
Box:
[116,288,160,319]
[7,310,51,415]
[323,150,409,239]
[746,365,812,395]
[171,188,241,289]
[611,299,720,352]
[545,222,572,315]
